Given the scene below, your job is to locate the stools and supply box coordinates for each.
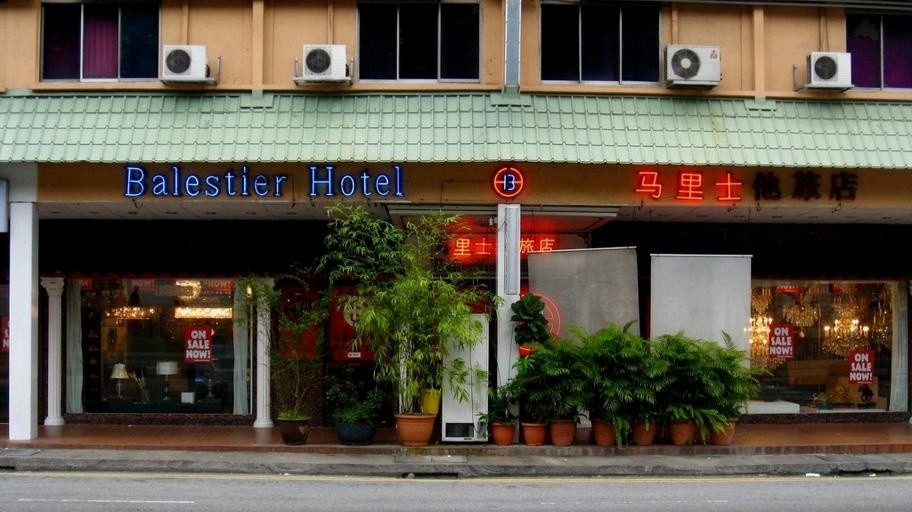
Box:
[197,358,219,403]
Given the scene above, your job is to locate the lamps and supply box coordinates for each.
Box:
[156,361,179,400]
[110,363,129,400]
[746,283,892,360]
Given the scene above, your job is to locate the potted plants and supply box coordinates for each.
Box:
[547,340,580,447]
[707,330,776,445]
[476,386,518,444]
[669,335,730,444]
[230,256,329,442]
[569,319,639,445]
[506,354,548,445]
[349,208,507,447]
[626,330,685,445]
[311,204,417,447]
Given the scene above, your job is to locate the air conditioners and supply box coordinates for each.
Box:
[663,44,721,83]
[163,46,206,80]
[807,52,852,85]
[303,44,346,77]
